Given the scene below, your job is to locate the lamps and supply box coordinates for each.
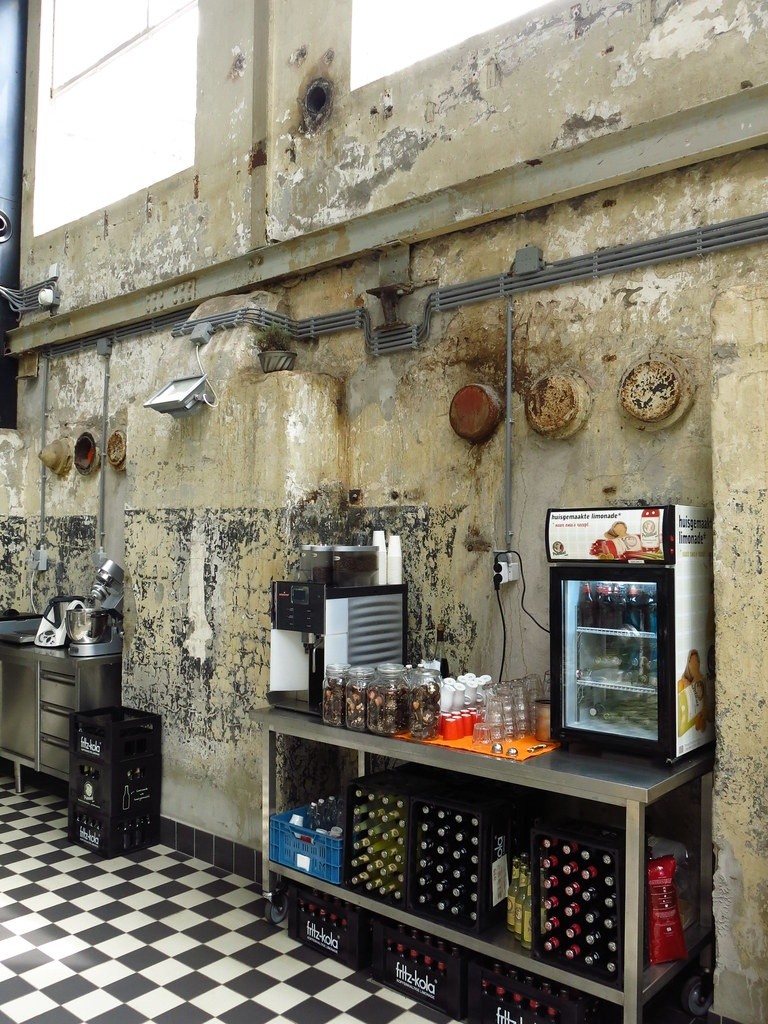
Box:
[143,375,215,420]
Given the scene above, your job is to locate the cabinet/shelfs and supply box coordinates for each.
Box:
[1,642,122,793]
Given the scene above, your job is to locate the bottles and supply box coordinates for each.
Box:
[581,584,656,632]
[323,663,443,740]
[594,637,657,689]
[76,766,151,849]
[482,963,598,1024]
[369,918,459,977]
[299,889,360,932]
[290,796,343,844]
[419,805,479,922]
[350,789,409,899]
[591,693,658,730]
[507,837,618,980]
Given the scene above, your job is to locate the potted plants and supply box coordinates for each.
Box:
[254,322,297,372]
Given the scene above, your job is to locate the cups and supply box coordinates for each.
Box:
[372,530,387,585]
[388,535,402,585]
[438,669,551,745]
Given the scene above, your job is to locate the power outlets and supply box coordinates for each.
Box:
[491,561,519,584]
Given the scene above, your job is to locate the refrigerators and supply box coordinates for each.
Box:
[545,505,715,764]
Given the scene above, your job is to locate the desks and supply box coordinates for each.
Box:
[248,705,713,1023]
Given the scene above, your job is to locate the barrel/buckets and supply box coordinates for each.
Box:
[65,609,109,643]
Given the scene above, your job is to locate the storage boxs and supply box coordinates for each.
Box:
[469,958,626,1023]
[286,884,367,975]
[530,813,624,986]
[407,778,517,926]
[343,764,441,909]
[268,797,346,887]
[373,917,468,1021]
[66,706,161,857]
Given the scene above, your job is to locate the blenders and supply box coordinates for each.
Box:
[68,560,123,656]
[266,581,409,716]
